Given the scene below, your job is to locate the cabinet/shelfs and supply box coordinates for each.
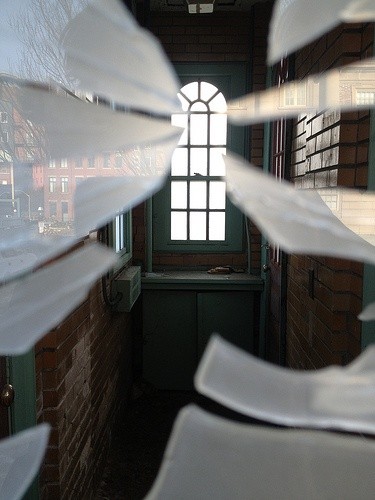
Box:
[140,270,267,380]
